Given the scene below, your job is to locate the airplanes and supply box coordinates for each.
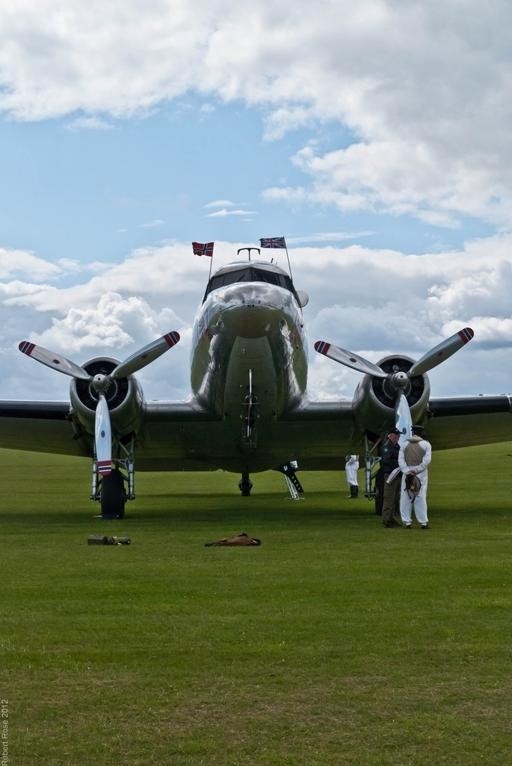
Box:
[0,232,511,524]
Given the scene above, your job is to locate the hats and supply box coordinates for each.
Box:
[411,425,424,431]
[388,428,404,434]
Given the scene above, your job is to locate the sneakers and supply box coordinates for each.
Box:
[348,494,357,498]
[385,519,430,529]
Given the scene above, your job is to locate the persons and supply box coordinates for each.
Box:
[398,424,433,528]
[344,454,359,497]
[381,427,403,527]
